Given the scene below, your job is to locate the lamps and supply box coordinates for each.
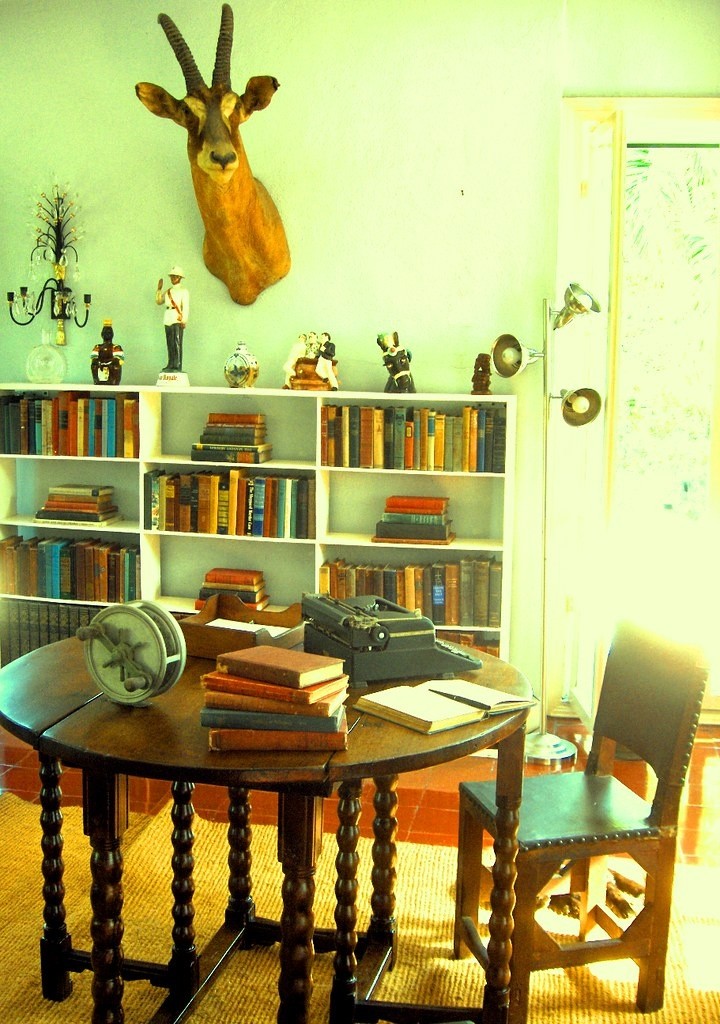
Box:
[7,180,91,346]
[491,281,603,766]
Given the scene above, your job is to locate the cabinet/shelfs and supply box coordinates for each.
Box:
[1,382,518,759]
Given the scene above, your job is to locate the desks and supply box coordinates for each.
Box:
[1,635,532,1024]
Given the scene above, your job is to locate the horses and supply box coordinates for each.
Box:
[376,331,416,393]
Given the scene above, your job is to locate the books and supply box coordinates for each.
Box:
[371,495,456,545]
[196,568,270,612]
[0,391,141,664]
[191,412,274,465]
[201,646,349,751]
[320,556,501,660]
[144,468,316,540]
[320,404,506,474]
[354,678,538,736]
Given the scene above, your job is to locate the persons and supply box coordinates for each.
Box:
[155,267,189,372]
[283,332,339,391]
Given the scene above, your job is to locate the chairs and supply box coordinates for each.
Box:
[450,619,706,1023]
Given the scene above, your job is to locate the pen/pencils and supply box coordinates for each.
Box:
[429,687,492,713]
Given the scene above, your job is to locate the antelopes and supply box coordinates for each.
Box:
[134,3,292,308]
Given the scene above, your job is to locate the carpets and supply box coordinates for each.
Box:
[0,789,720,1024]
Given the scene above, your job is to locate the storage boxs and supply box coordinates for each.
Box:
[176,591,312,661]
